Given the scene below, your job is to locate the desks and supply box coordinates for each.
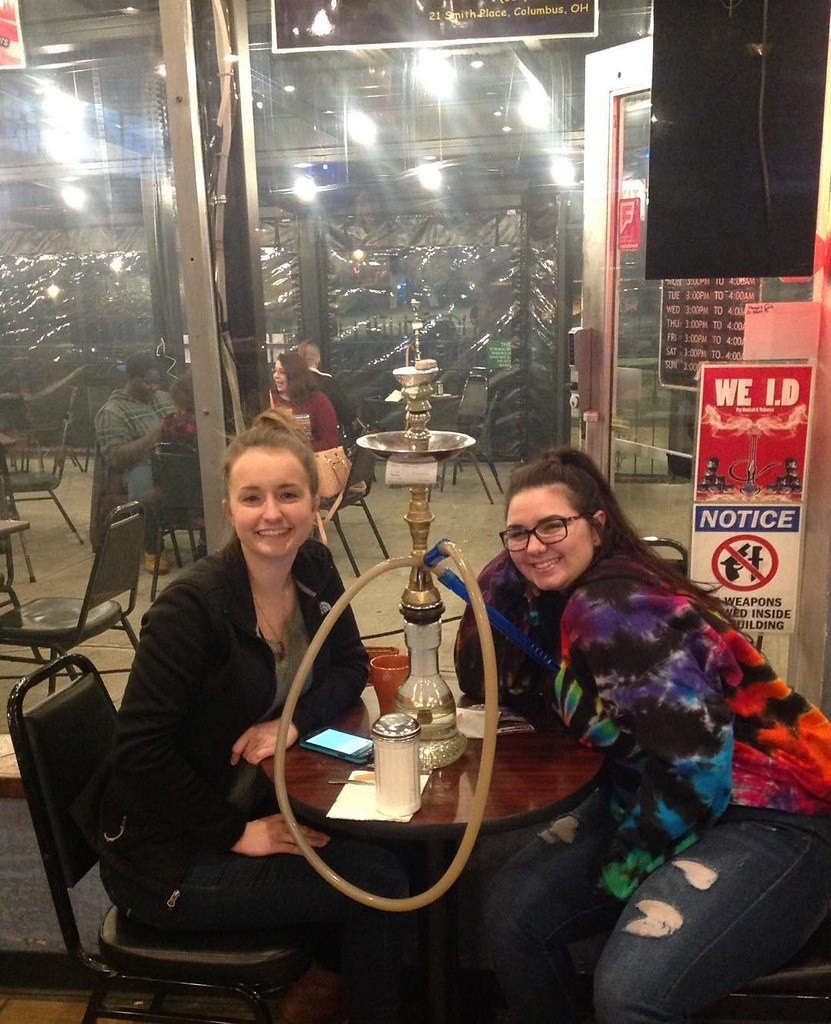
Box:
[366,390,462,488]
[261,680,608,1023]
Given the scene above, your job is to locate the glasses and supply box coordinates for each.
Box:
[498,516,584,552]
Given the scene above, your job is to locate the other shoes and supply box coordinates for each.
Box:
[145,549,169,575]
[197,544,207,558]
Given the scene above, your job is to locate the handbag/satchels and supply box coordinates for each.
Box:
[313,446,366,498]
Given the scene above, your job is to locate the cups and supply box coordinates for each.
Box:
[369,653,409,716]
[369,711,422,817]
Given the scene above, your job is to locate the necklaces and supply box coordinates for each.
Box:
[254,583,292,652]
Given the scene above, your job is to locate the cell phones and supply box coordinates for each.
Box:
[299,726,374,765]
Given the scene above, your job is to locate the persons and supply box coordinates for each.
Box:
[299,340,358,423]
[267,352,338,453]
[94,354,206,574]
[453,446,831,1024]
[100,413,424,1023]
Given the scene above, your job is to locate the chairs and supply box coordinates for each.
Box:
[542,889,831,1024]
[312,428,390,577]
[0,370,304,1023]
[427,374,503,505]
